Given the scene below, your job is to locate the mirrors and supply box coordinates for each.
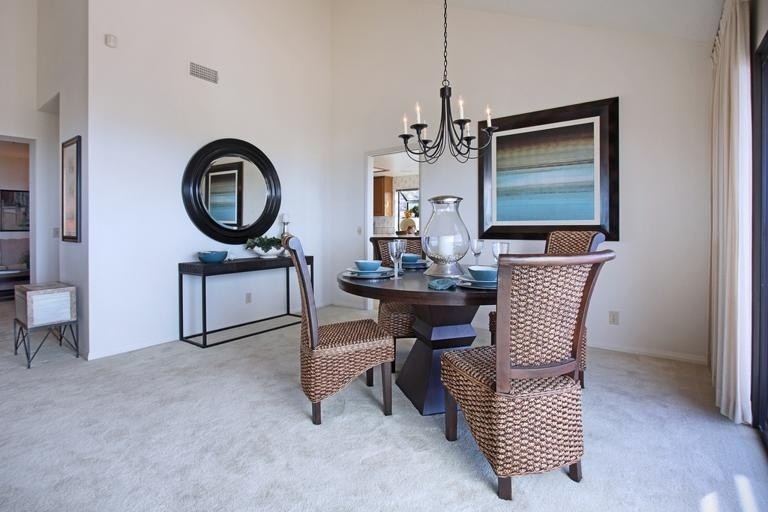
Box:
[181,136,281,242]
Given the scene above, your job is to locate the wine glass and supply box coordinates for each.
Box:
[388,238,407,281]
[470,238,486,264]
[490,240,512,259]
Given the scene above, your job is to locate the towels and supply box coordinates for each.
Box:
[428,276,456,291]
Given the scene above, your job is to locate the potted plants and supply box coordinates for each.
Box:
[245,235,285,259]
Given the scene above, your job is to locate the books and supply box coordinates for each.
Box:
[0,262,28,274]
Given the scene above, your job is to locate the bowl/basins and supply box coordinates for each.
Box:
[400,251,420,262]
[467,265,500,280]
[354,260,383,271]
[197,251,229,264]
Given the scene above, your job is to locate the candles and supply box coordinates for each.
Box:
[457,95,465,119]
[414,101,422,123]
[283,213,288,221]
[485,106,493,127]
[402,114,408,134]
[465,122,471,136]
[422,121,429,140]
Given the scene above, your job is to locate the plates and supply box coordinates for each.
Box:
[401,260,429,270]
[342,266,393,281]
[457,276,499,291]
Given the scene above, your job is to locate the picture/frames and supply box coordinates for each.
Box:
[0,188,31,233]
[204,160,243,228]
[60,135,82,243]
[477,95,622,242]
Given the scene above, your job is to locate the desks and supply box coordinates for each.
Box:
[177,254,313,349]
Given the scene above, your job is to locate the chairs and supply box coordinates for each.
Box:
[278,233,397,422]
[372,233,440,375]
[439,248,624,503]
[488,229,609,390]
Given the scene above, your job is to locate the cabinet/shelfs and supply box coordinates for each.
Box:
[371,175,393,218]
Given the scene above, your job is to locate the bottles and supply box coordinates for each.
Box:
[421,195,471,278]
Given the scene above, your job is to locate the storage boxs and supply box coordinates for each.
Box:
[13,278,78,328]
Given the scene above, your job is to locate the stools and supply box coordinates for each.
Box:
[12,317,81,368]
